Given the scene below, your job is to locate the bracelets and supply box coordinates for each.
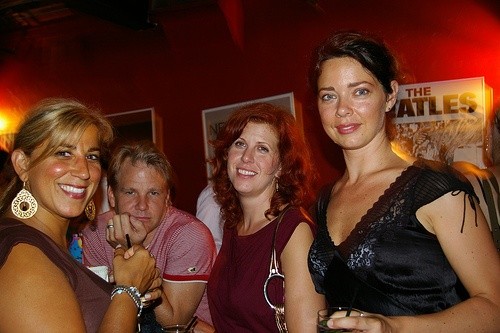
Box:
[110,285,145,320]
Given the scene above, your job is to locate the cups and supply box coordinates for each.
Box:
[316,307,364,333]
[162,324,192,333]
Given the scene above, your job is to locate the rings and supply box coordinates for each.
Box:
[106,225,115,228]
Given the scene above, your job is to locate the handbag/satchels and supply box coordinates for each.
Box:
[263,202,289,333]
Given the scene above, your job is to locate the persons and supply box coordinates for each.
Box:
[206,104,327,333]
[460,108,500,254]
[81,140,218,333]
[0,145,227,264]
[307,33,500,333]
[0,97,164,333]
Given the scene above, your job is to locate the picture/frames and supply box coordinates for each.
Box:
[202,92,295,184]
